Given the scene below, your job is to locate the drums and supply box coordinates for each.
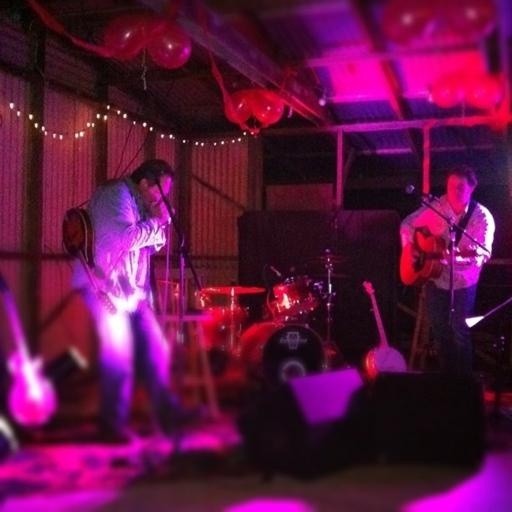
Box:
[200,307,252,356]
[239,320,324,382]
[265,276,325,324]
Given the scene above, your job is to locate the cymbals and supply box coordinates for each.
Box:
[200,285,265,295]
[158,278,180,286]
[158,312,207,322]
[311,273,348,280]
[302,256,350,264]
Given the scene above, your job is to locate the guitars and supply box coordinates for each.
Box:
[399,228,487,287]
[1,275,60,428]
[361,280,408,381]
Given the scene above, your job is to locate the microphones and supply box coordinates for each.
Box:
[404,184,440,203]
[269,264,282,277]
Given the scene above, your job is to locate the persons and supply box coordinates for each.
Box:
[69,157,202,442]
[398,164,496,363]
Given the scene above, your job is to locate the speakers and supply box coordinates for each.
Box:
[237,367,371,478]
[472,261,512,380]
[371,372,485,465]
